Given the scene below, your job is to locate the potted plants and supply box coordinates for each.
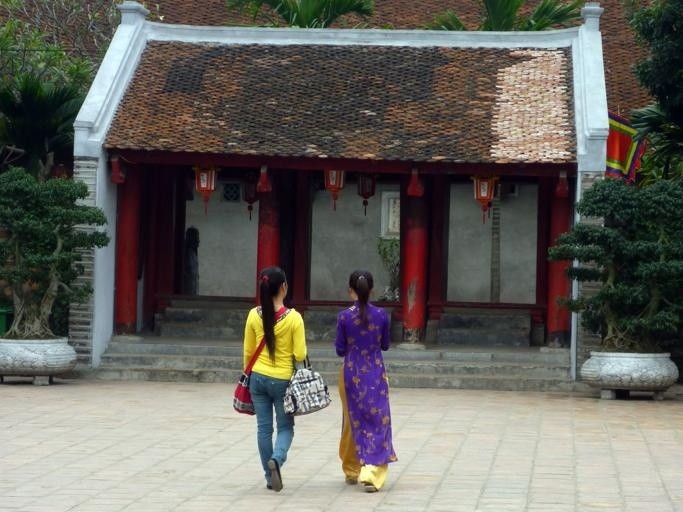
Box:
[546,174,682,399]
[0,164,110,386]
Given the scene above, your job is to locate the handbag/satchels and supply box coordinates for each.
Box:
[283,353,332,417]
[233,373,258,415]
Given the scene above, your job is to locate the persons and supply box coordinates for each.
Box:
[242,265,307,493]
[335,270,397,492]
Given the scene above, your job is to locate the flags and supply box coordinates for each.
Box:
[605,110,649,186]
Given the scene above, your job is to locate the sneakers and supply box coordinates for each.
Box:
[267,458,284,492]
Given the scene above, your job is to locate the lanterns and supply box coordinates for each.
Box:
[357,171,376,217]
[242,172,260,221]
[486,175,502,218]
[325,170,346,210]
[471,173,494,225]
[193,165,221,214]
